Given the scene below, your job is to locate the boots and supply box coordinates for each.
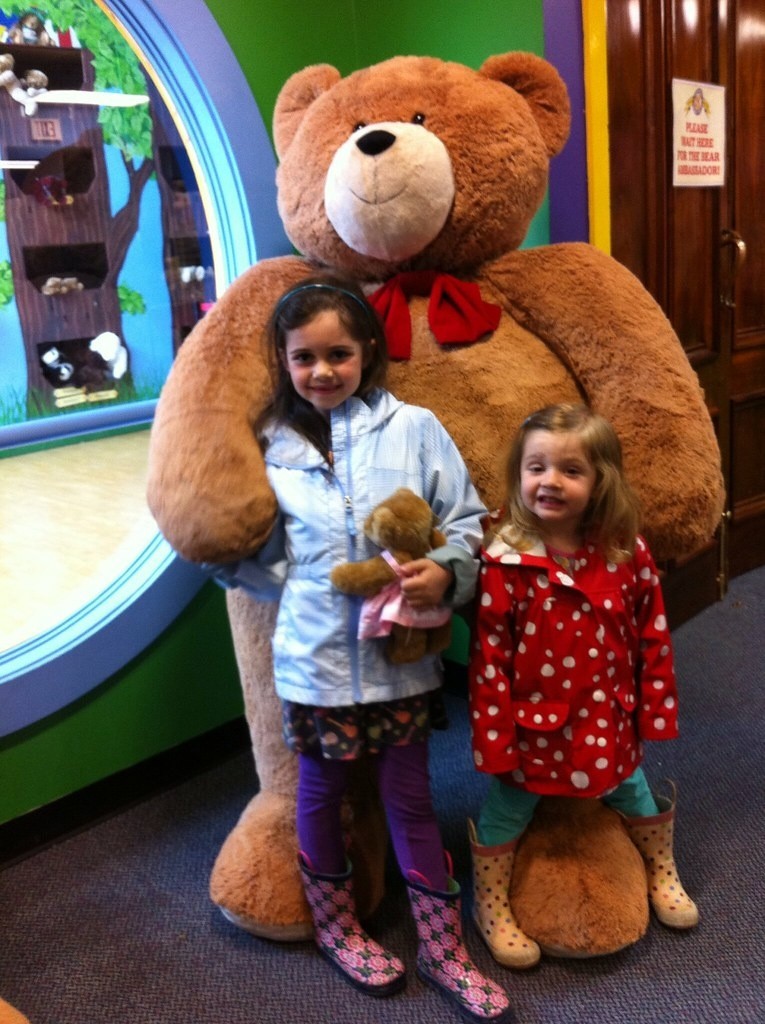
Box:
[612,774,699,929]
[467,817,541,969]
[296,849,407,997]
[404,849,514,1024]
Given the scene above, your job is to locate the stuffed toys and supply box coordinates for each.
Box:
[331,488,452,669]
[144,46,723,959]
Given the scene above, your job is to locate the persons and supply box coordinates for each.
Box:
[467,401,699,969]
[193,273,511,1024]
